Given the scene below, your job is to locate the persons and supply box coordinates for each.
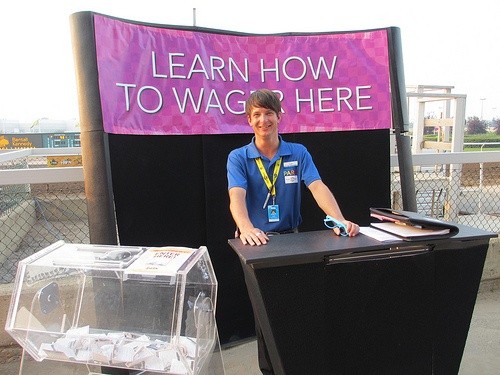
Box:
[227,87,360,375]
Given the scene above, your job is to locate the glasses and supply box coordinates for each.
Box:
[324,215,349,236]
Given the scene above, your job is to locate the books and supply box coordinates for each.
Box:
[370,207,459,242]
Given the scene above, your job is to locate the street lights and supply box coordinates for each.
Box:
[480,96,486,123]
